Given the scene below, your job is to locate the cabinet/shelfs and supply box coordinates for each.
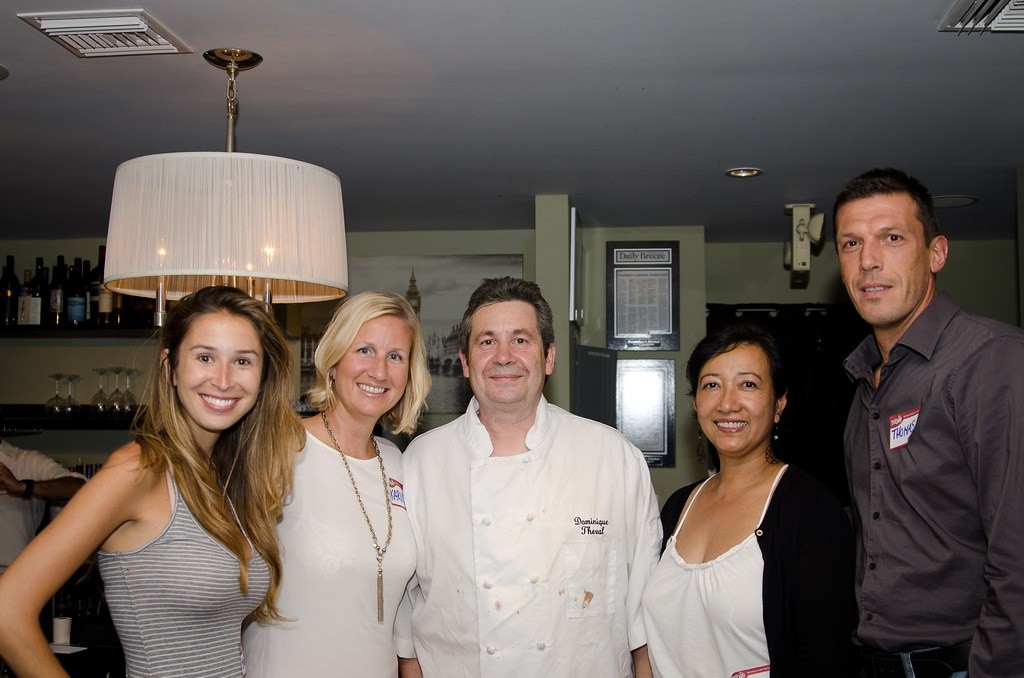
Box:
[0,323,159,429]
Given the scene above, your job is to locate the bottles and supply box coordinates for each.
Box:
[0,243,128,330]
[47,456,104,485]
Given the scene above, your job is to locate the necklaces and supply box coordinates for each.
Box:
[321,411,392,621]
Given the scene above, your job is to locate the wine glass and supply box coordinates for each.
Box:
[45,372,82,417]
[91,367,142,415]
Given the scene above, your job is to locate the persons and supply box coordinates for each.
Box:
[0,436,88,577]
[832,168,1024,678]
[392,276,664,678]
[242,289,432,678]
[642,325,858,678]
[0,284,308,678]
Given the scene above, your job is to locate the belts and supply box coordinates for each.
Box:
[864,639,971,678]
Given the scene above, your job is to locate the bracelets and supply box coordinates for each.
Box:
[22,480,34,500]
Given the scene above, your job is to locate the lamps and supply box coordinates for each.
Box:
[103,48,349,326]
[784,203,825,272]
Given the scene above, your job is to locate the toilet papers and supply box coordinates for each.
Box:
[53,618,72,645]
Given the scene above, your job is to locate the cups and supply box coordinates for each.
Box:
[53,616,71,645]
